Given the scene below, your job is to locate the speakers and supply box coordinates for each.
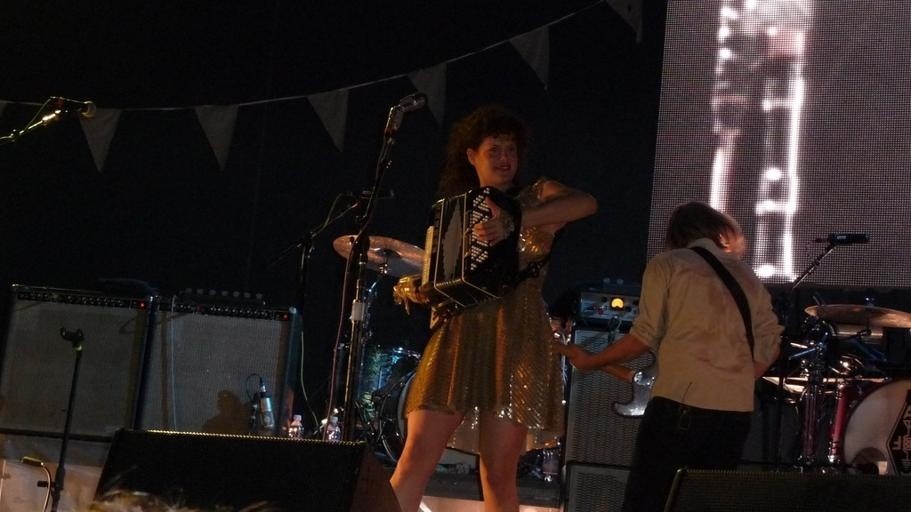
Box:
[1,298,148,442]
[85,426,401,512]
[139,312,288,435]
[662,467,911,511]
[563,327,655,463]
[568,467,629,511]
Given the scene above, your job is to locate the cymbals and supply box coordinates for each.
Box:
[805,304,911,329]
[334,233,426,278]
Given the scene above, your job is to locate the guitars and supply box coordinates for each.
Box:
[558,341,659,417]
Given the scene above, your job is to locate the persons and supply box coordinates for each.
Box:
[389,106,601,509]
[570,202,787,512]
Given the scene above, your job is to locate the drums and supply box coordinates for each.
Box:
[842,379,910,474]
[380,372,479,475]
[359,339,419,409]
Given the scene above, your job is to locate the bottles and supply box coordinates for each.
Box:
[326,416,341,441]
[542,434,562,476]
[289,414,304,437]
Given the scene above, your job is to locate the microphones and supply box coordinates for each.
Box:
[399,92,428,110]
[253,386,277,429]
[817,233,869,244]
[49,95,97,118]
